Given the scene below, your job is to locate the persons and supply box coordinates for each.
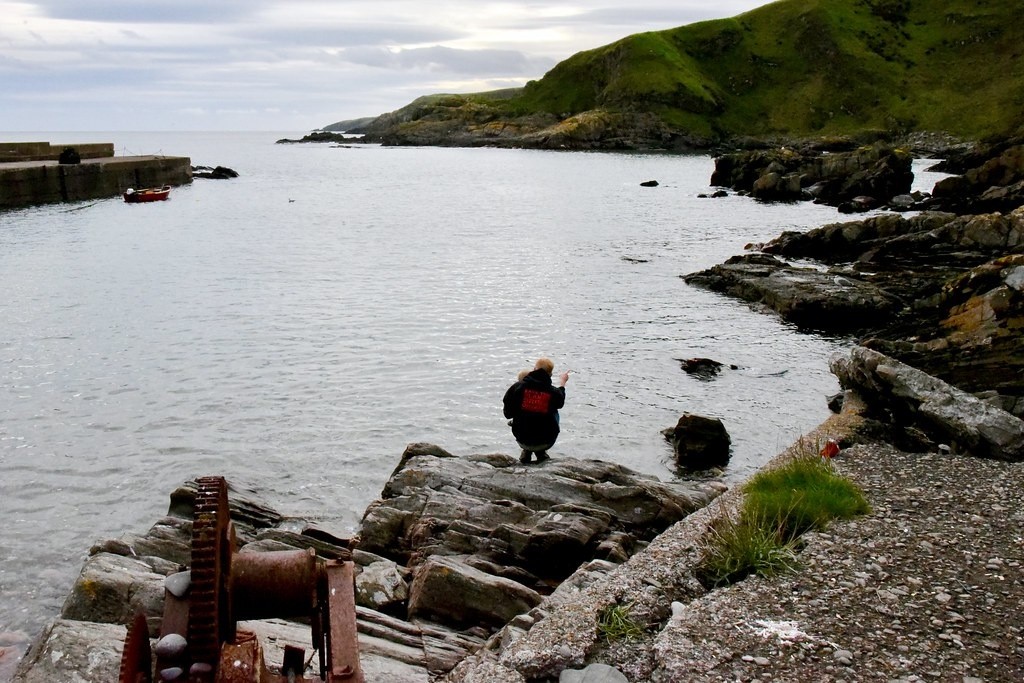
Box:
[503,359,571,461]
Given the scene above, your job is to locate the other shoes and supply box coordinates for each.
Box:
[519,450,532,462]
[508,420,513,427]
[537,453,551,461]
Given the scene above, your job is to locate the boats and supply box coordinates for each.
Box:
[123,185,172,203]
